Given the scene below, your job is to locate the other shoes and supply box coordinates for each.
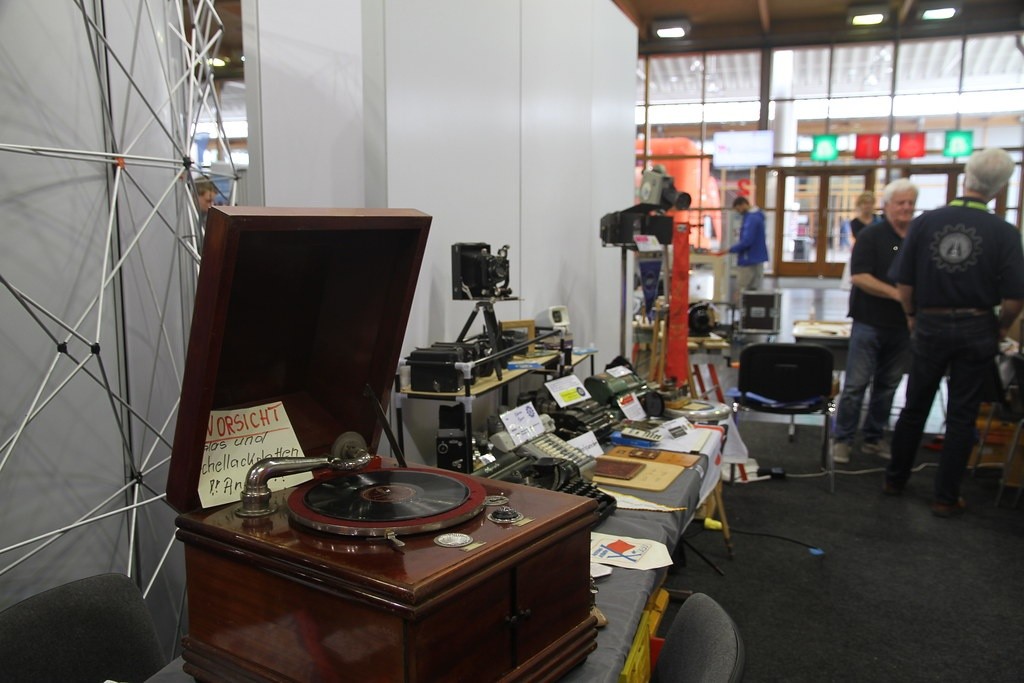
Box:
[861,441,891,459]
[828,440,852,463]
[886,471,907,489]
[931,497,954,516]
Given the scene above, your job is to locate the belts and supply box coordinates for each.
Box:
[917,308,990,316]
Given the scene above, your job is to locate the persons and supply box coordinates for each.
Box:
[847,192,884,246]
[719,197,768,308]
[189,176,218,229]
[832,148,1024,517]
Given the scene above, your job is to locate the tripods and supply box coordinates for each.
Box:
[455,297,508,383]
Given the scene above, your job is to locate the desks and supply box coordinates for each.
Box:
[689,252,732,307]
[786,320,951,446]
[550,424,736,683]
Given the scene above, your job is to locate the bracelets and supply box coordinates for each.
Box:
[906,313,914,317]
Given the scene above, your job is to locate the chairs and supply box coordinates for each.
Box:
[647,592,746,683]
[725,342,837,491]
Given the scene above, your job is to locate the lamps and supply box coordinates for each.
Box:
[651,18,692,39]
[846,2,890,28]
[916,0,964,22]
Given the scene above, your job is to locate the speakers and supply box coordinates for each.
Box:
[435,428,468,472]
